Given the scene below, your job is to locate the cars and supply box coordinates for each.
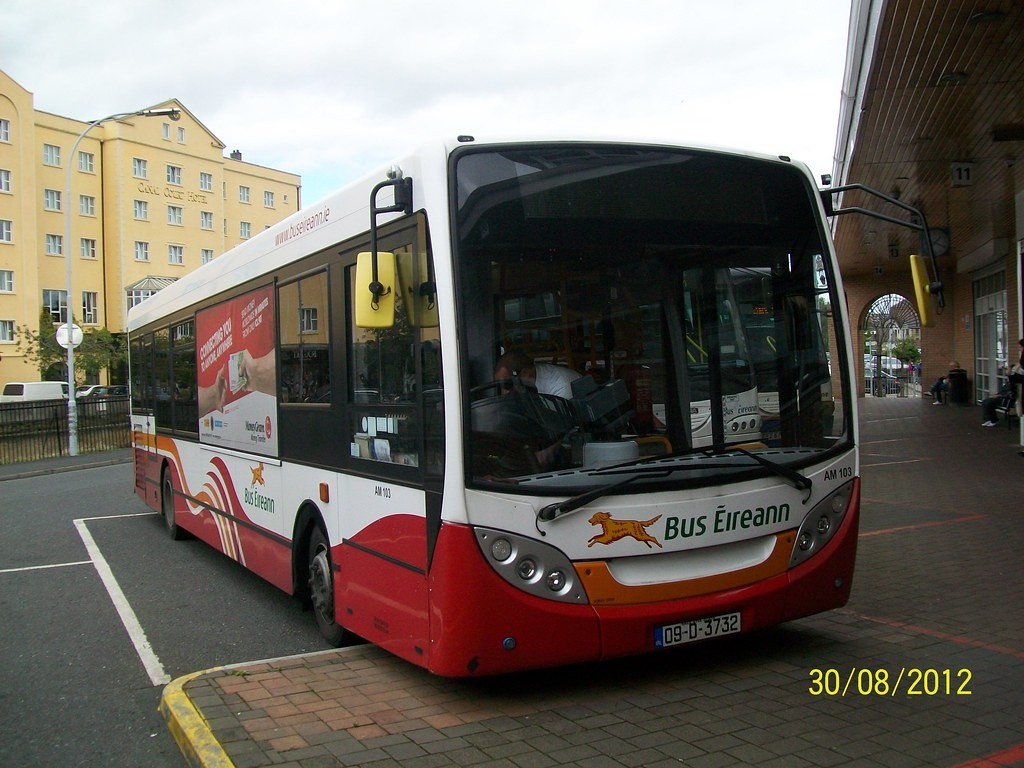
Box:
[864,353,910,380]
[353,388,381,405]
[77,383,129,403]
[424,389,444,412]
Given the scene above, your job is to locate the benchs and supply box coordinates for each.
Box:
[996,383,1022,431]
[944,369,969,405]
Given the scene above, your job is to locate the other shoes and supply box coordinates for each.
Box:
[933,401,942,404]
[923,392,932,396]
[987,422,1000,426]
[982,421,991,426]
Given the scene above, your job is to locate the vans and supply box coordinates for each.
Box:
[1,380,76,401]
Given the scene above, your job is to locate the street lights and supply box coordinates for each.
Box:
[65,107,188,457]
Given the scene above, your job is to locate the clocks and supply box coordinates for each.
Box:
[919,225,951,258]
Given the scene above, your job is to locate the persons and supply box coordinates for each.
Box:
[494,351,584,475]
[923,361,960,405]
[916,362,922,377]
[239,347,276,397]
[198,364,229,420]
[981,362,1016,426]
[908,361,914,383]
[1011,338,1024,416]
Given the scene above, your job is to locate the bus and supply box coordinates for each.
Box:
[124,133,947,691]
[732,265,835,449]
[645,265,762,447]
